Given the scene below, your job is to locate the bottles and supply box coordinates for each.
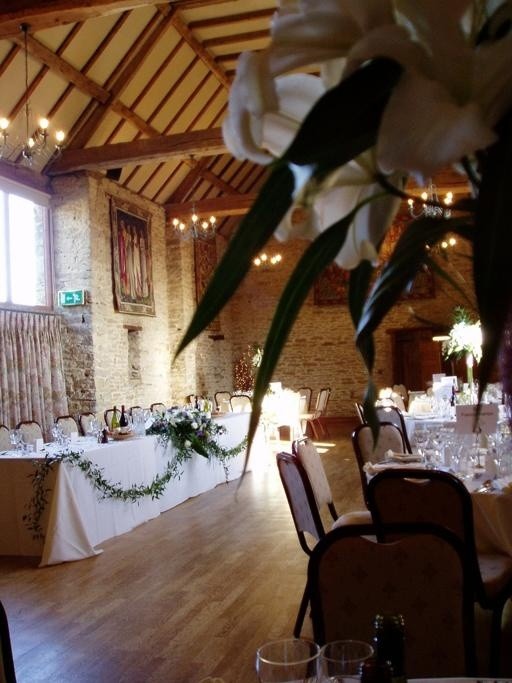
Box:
[187,396,208,412]
[119,405,128,428]
[110,405,120,431]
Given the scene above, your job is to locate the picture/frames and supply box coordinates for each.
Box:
[314,213,436,306]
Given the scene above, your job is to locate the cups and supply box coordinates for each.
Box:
[374,615,406,683]
[256,639,321,683]
[321,641,373,683]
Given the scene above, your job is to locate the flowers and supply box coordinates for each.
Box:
[172,1,512,492]
[433,306,484,388]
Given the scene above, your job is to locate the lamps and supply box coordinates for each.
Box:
[0,24,65,168]
[171,200,216,241]
[407,184,453,219]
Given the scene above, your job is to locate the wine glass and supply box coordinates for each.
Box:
[8,418,102,452]
[409,429,512,482]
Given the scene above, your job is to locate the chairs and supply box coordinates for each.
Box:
[351,422,406,511]
[354,383,410,429]
[275,451,325,639]
[292,437,373,530]
[367,467,511,675]
[307,523,477,675]
